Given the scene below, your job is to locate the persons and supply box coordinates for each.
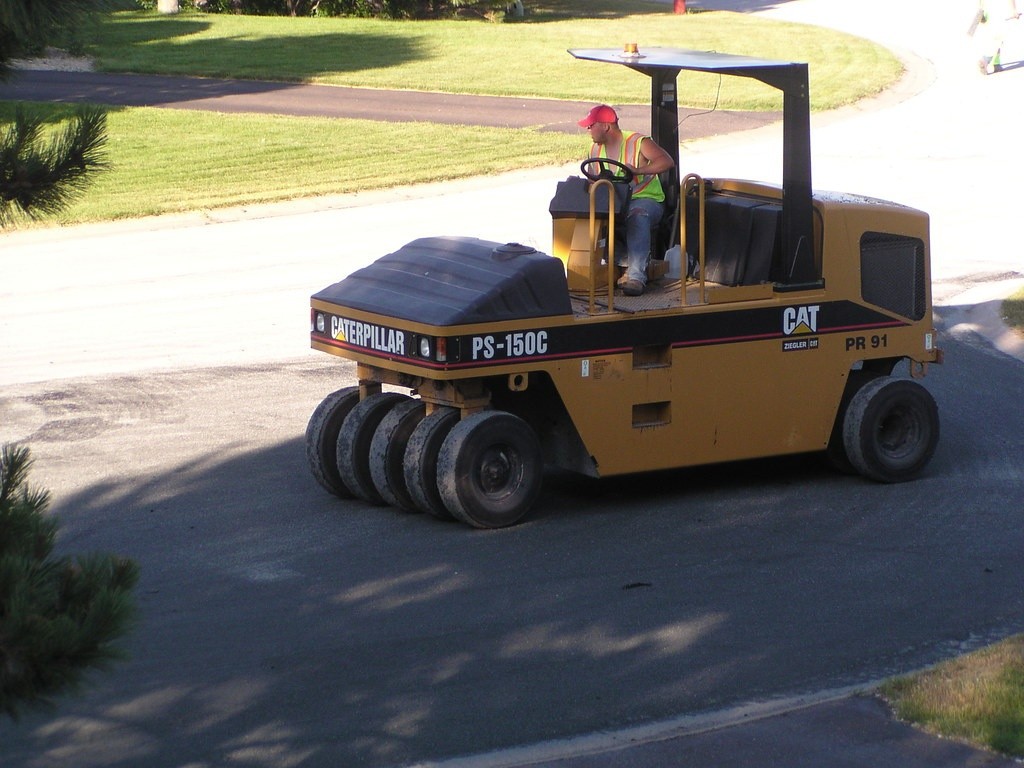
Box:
[970,0,1022,75]
[578,104,674,295]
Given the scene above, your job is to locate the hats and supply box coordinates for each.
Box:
[578,104,618,127]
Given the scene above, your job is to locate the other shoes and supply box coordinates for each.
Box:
[617,268,629,289]
[623,279,644,296]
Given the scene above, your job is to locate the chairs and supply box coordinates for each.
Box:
[652,162,680,229]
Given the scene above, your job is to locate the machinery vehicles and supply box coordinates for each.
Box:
[304,44,943,530]
